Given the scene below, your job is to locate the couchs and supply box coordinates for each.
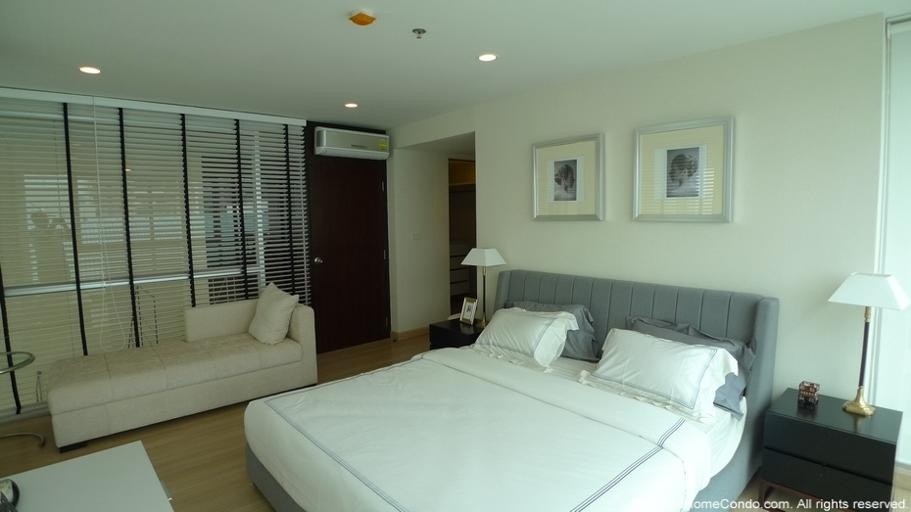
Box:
[47,282,318,450]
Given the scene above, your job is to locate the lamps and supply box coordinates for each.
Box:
[828,272,907,416]
[460,248,506,328]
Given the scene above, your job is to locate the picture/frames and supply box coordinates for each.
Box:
[459,296,479,326]
[633,116,735,223]
[532,133,606,222]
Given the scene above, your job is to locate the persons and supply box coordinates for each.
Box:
[554,164,577,200]
[667,155,699,197]
[31,211,70,286]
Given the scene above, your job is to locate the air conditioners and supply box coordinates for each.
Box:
[314,126,390,161]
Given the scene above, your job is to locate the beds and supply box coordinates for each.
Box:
[243,269,780,512]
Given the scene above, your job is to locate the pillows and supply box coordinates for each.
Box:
[503,301,601,362]
[577,328,738,423]
[247,282,299,345]
[625,316,757,420]
[470,307,580,374]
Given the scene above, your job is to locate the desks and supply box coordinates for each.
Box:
[0,351,46,447]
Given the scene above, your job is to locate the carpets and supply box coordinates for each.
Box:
[0,440,176,512]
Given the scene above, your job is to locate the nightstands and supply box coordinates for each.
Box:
[429,318,489,349]
[758,388,903,512]
